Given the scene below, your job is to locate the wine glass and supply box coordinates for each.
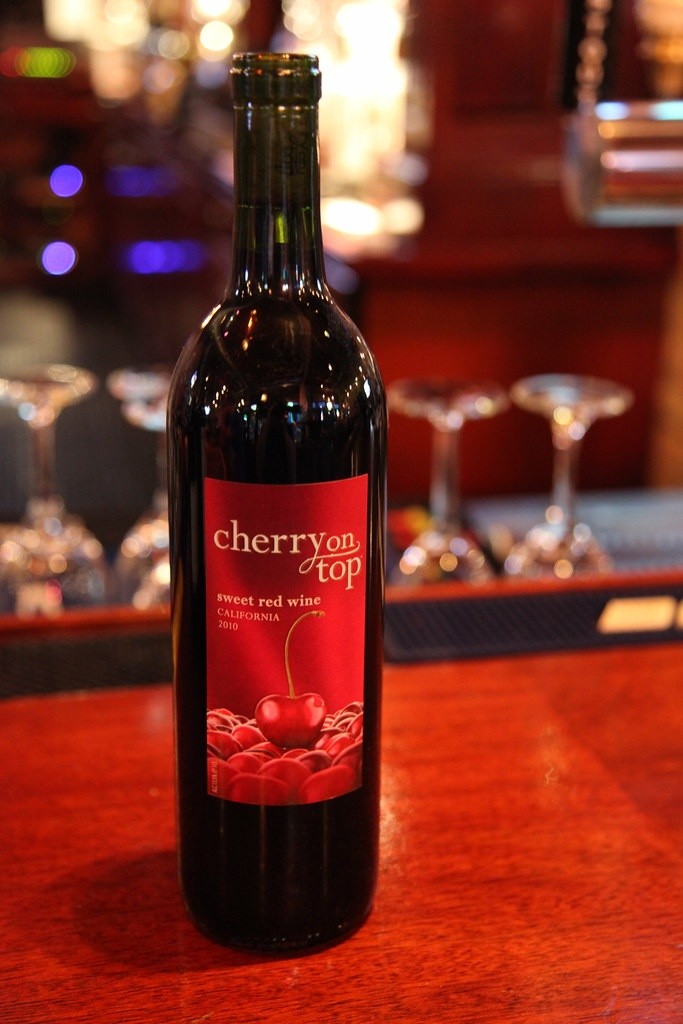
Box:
[0,365,169,615]
[381,373,633,588]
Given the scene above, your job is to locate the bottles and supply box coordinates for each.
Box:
[155,53,387,962]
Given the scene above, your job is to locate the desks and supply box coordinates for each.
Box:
[0,568,682,1024]
